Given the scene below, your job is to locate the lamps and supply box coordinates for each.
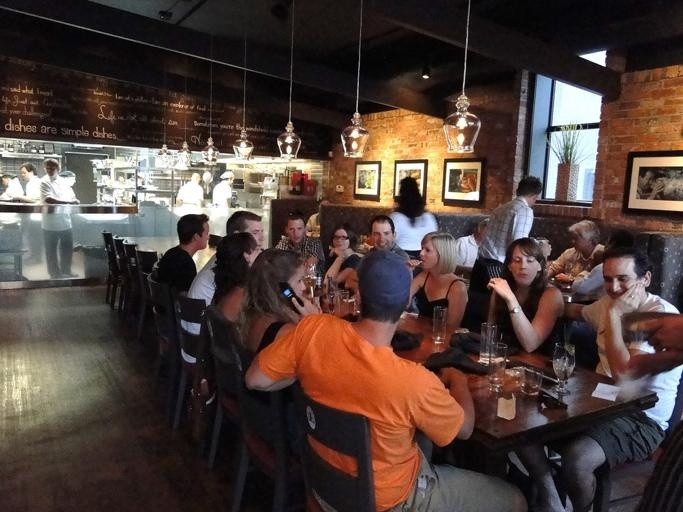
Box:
[233,1,255,167]
[340,1,368,158]
[278,1,301,160]
[202,66,220,167]
[156,94,172,159]
[441,1,481,154]
[178,70,192,168]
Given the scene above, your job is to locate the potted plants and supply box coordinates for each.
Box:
[546,118,596,201]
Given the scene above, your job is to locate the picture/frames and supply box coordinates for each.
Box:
[392,159,427,202]
[442,157,486,207]
[622,150,683,220]
[354,161,381,200]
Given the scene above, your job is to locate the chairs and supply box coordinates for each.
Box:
[102,229,118,309]
[594,443,663,512]
[206,319,285,509]
[174,296,213,453]
[298,393,374,512]
[134,247,157,337]
[146,273,178,380]
[113,233,131,315]
[119,240,139,313]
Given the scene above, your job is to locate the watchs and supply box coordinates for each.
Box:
[507,304,524,317]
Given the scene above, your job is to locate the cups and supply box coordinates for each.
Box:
[432,306,448,344]
[488,343,508,393]
[337,290,349,317]
[327,274,339,315]
[520,366,543,395]
[552,342,576,396]
[341,299,355,316]
[480,322,497,362]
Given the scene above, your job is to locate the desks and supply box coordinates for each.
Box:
[321,295,659,464]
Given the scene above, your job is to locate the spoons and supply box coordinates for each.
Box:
[505,368,568,406]
[512,366,558,384]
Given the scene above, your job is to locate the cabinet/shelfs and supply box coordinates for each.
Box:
[89,149,226,213]
[1,149,64,161]
[269,196,319,247]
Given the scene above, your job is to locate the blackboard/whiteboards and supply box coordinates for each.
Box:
[2,51,350,158]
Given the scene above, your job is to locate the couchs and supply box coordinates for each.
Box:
[319,205,683,311]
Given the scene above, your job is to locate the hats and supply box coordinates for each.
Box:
[357,250,411,304]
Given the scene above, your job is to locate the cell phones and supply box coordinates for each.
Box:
[280,283,303,315]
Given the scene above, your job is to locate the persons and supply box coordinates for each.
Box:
[244,248,526,510]
[176,174,204,210]
[304,200,329,238]
[408,232,469,328]
[489,236,565,366]
[274,208,324,268]
[181,209,264,365]
[635,168,663,200]
[19,163,46,264]
[210,232,257,400]
[150,213,210,339]
[1,173,23,234]
[480,176,542,282]
[571,230,629,303]
[622,311,683,509]
[212,173,237,214]
[325,224,365,290]
[650,173,666,199]
[370,214,407,266]
[571,236,680,510]
[452,216,488,278]
[42,159,76,280]
[229,249,321,445]
[390,178,441,250]
[544,220,607,283]
